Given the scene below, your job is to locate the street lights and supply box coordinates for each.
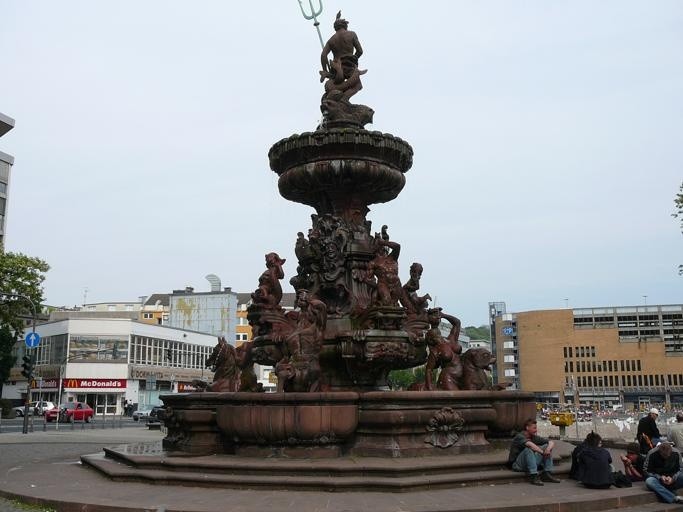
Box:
[641,295,648,305]
[563,297,568,308]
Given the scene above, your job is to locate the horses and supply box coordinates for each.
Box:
[191,337,242,392]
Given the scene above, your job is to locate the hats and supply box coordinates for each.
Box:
[648,408,658,416]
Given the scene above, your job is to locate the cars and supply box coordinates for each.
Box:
[12,399,55,417]
[536,402,683,425]
[131,404,157,421]
[44,401,96,425]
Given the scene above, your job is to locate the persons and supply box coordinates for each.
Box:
[569,432,592,480]
[575,433,613,489]
[637,408,661,454]
[123,399,128,415]
[643,441,682,504]
[666,411,683,459]
[307,239,351,315]
[423,310,465,391]
[127,399,133,417]
[356,237,415,311]
[317,10,373,124]
[508,417,561,486]
[619,444,647,482]
[71,402,82,409]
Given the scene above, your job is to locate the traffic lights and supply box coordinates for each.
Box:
[166,347,172,360]
[20,354,34,382]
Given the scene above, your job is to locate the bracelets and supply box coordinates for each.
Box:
[627,460,632,465]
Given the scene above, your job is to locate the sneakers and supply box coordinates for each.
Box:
[675,497,683,503]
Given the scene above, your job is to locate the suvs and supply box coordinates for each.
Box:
[148,403,166,426]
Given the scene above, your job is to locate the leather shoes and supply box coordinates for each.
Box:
[541,471,560,483]
[531,475,544,486]
